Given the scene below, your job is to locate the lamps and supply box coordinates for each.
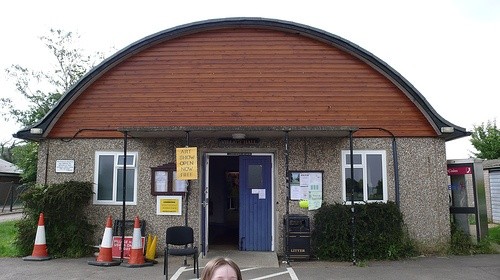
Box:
[232,134,246,139]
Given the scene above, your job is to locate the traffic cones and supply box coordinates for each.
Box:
[145,233,153,254]
[21,213,51,261]
[143,235,158,264]
[120,216,153,268]
[88,214,121,267]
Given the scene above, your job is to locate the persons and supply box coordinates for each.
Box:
[200,257,242,280]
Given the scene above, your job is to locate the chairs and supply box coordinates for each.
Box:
[164,226,199,280]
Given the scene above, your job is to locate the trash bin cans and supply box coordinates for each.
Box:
[283,214,311,260]
